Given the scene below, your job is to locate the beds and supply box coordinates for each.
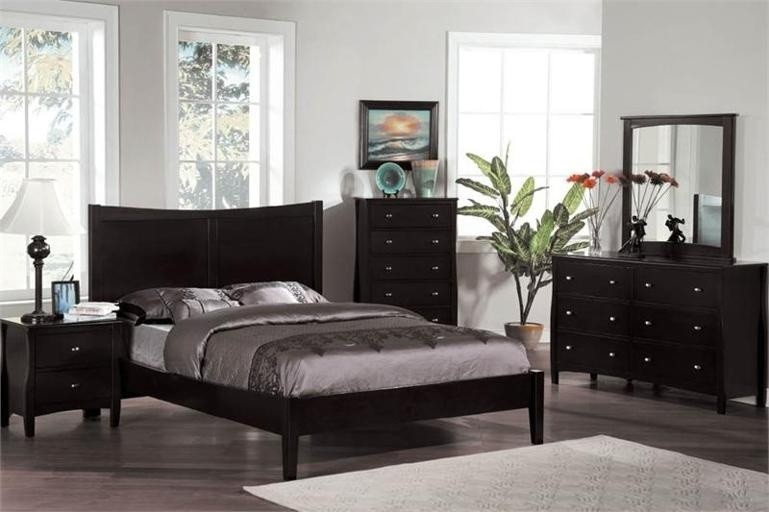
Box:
[88,197,544,479]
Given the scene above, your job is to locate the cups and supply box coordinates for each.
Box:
[410,159,440,198]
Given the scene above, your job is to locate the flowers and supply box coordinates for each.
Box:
[570,167,627,248]
[629,166,677,222]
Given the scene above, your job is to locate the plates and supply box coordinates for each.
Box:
[375,162,406,195]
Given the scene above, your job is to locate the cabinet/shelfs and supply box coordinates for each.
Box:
[352,194,459,327]
[547,249,769,419]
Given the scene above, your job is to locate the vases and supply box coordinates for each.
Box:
[587,236,602,255]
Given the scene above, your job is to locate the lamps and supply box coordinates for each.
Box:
[2,177,87,321]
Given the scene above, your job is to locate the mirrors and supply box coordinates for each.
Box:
[615,109,737,262]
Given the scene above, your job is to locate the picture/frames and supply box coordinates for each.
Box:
[50,276,81,315]
[358,99,439,170]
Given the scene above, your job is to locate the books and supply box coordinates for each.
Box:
[68,300,115,316]
[62,311,118,323]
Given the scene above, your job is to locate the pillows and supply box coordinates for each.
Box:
[155,286,242,327]
[113,286,174,326]
[226,280,330,310]
[173,298,240,327]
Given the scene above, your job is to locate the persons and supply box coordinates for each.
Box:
[665,214,687,243]
[626,214,648,253]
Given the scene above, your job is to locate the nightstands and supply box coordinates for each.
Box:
[0,314,131,439]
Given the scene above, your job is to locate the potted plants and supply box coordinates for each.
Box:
[456,147,598,350]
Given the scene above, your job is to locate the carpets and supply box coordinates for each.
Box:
[242,434,769,511]
